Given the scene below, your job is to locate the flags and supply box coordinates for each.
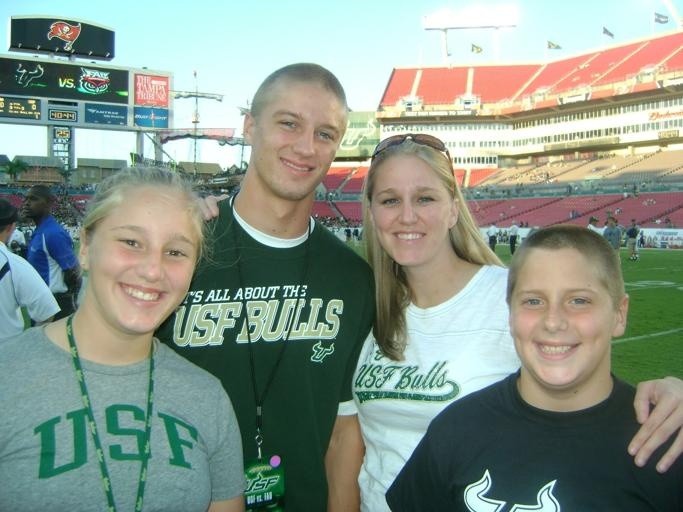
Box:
[603,27,613,38]
[548,41,562,49]
[655,13,669,24]
[472,45,482,53]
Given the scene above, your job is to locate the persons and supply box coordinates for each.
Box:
[386,223,683,512]
[155,64,376,511]
[199,134,683,511]
[1,169,245,511]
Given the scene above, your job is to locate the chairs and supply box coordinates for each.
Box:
[1,190,90,224]
[309,164,368,220]
[453,147,682,229]
[381,63,538,116]
[538,33,682,93]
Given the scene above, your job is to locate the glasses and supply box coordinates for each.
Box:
[370,133,454,181]
